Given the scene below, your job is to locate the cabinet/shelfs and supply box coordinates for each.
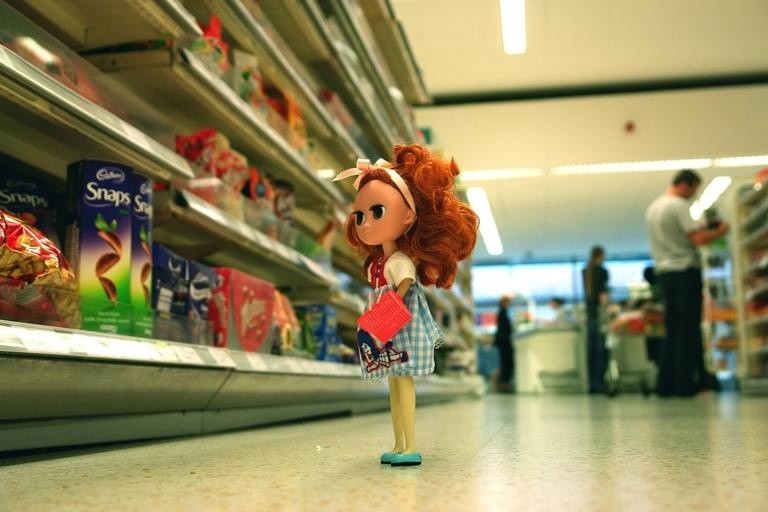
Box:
[0,0,483,462]
[730,174,768,398]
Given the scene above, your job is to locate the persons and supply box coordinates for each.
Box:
[582,246,611,395]
[491,294,513,392]
[330,139,480,469]
[645,168,732,397]
[540,298,576,328]
